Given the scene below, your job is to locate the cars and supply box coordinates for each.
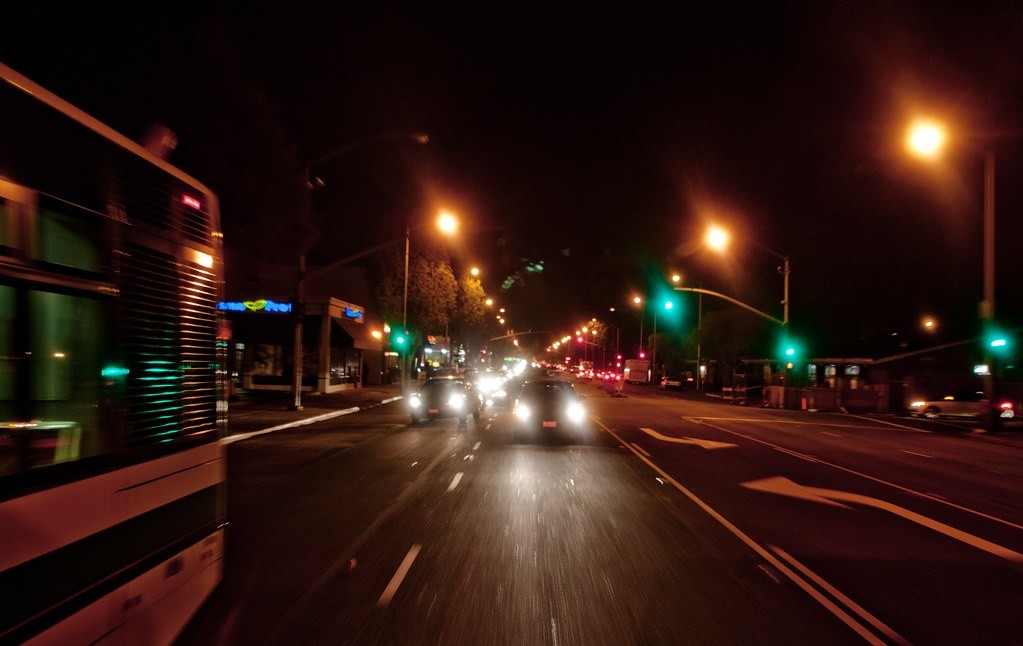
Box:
[472,356,553,409]
[663,375,685,392]
[410,374,482,425]
[513,377,588,443]
[907,389,991,419]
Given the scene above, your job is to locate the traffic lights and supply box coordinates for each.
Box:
[784,344,798,360]
[395,335,406,347]
[990,336,1007,354]
[659,293,677,316]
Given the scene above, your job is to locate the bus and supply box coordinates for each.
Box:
[0,59,227,646]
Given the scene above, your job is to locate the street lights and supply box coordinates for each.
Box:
[706,220,789,382]
[906,114,997,427]
